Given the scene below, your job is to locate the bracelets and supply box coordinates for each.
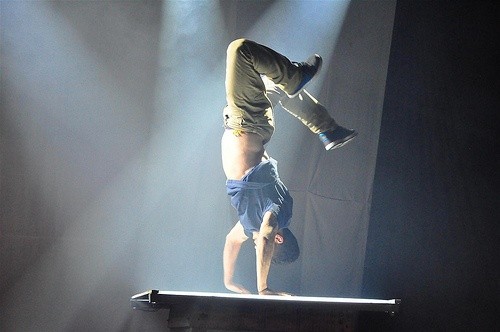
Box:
[258,288,269,293]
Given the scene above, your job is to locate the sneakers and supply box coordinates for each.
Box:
[285,54,322,98]
[319,125,359,151]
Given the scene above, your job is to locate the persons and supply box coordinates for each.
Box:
[221,39,359,297]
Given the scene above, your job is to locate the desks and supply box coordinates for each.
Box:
[130,288,402,332]
